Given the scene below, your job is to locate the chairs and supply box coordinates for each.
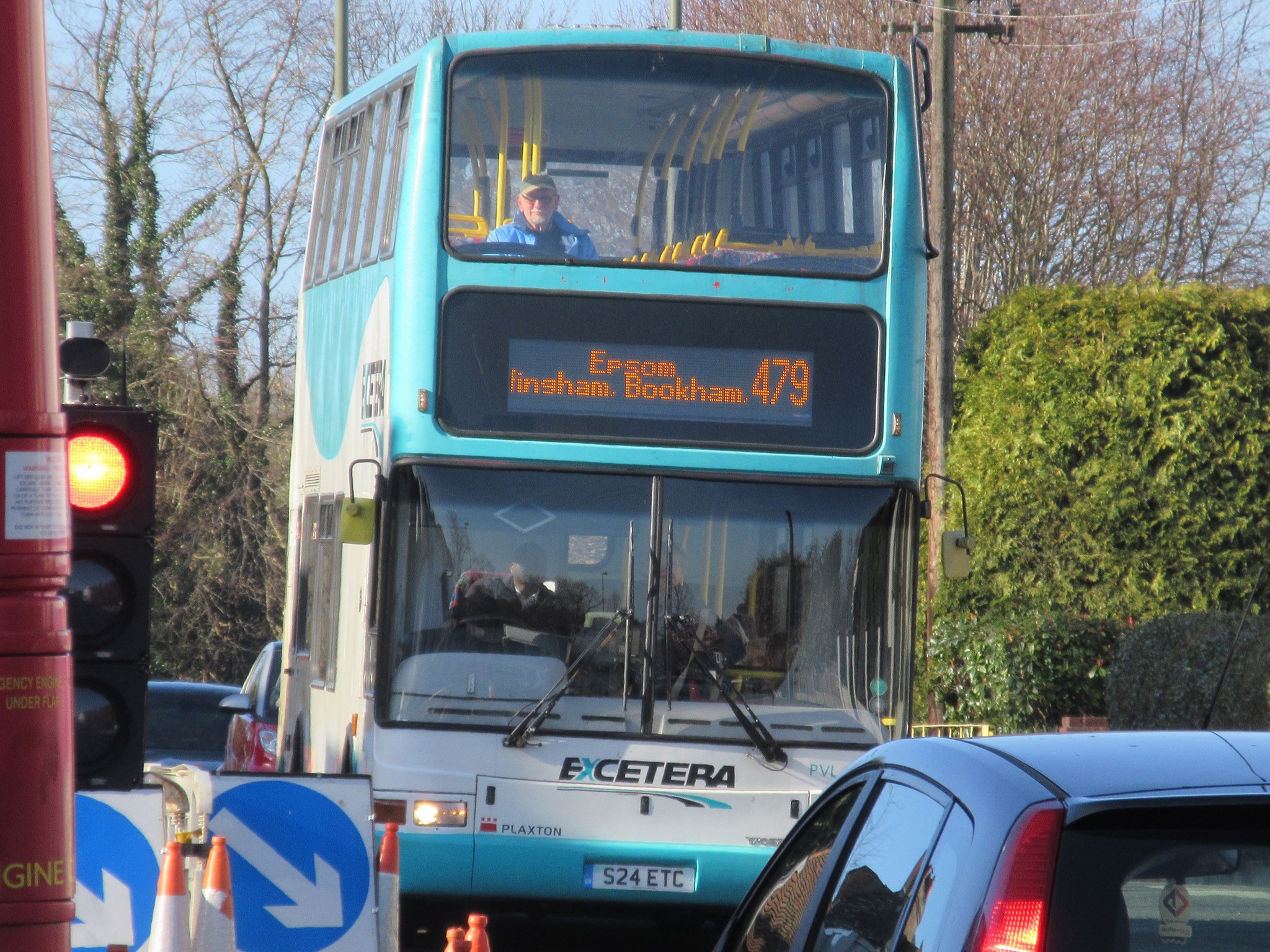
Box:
[445,564,792,701]
[444,209,886,279]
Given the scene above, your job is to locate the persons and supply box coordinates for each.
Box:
[612,540,699,618]
[703,613,721,646]
[483,173,600,261]
[458,540,574,649]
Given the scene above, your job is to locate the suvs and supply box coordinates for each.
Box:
[217,641,285,776]
[703,728,1269,952]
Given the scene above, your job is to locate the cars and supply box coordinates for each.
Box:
[144,677,243,780]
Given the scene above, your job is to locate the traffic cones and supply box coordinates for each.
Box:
[188,837,238,952]
[143,841,197,952]
[374,819,403,952]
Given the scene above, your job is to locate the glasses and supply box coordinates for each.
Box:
[523,194,554,203]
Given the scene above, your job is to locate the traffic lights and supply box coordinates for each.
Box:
[65,404,164,795]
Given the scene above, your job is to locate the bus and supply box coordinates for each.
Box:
[273,27,975,930]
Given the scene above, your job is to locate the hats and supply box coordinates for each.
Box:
[519,174,556,195]
